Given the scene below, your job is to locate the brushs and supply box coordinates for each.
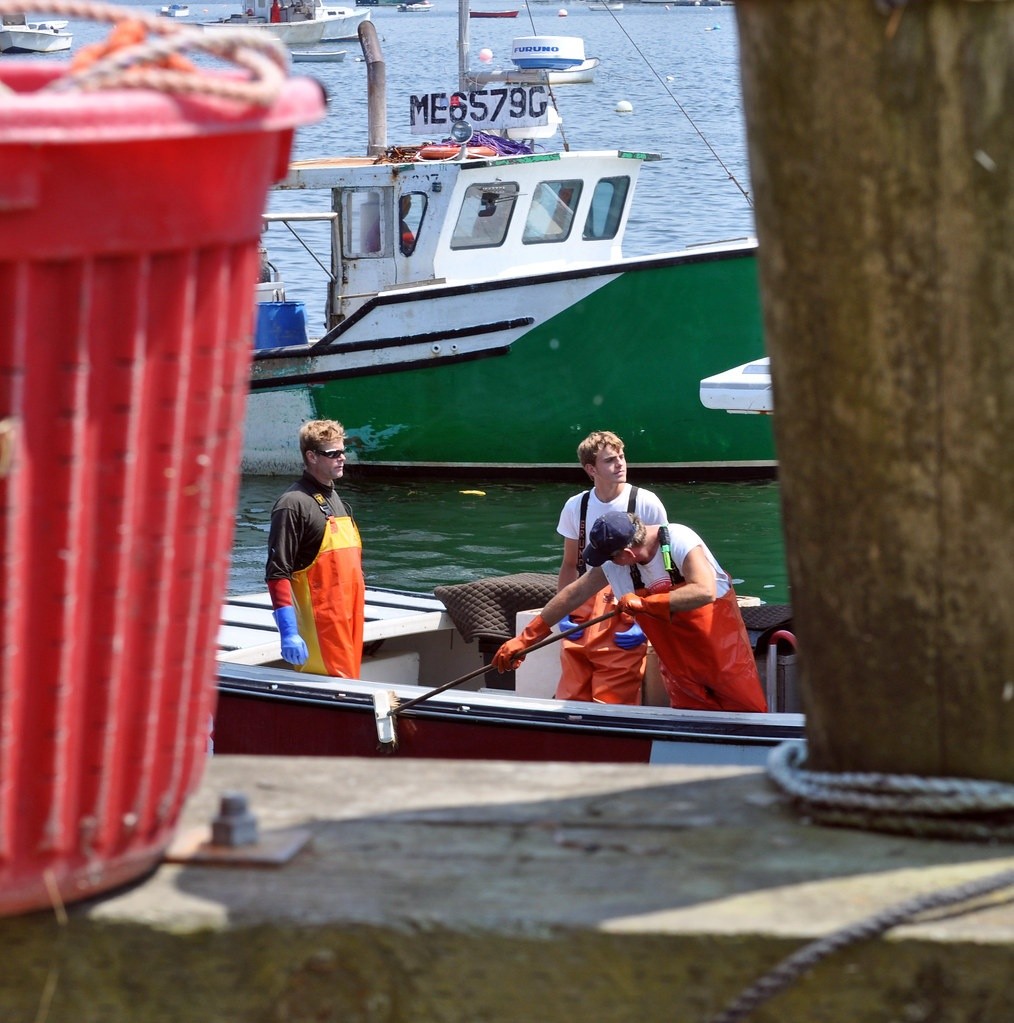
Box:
[371,609,628,751]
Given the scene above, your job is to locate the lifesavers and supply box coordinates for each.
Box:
[419,145,498,160]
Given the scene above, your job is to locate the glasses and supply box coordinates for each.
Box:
[312,449,345,459]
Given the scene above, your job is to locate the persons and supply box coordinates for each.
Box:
[554,431,669,706]
[264,420,365,678]
[368,194,415,257]
[491,511,770,712]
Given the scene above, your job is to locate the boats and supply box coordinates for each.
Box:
[397,3,435,12]
[588,2,624,11]
[0,21,73,53]
[209,576,806,770]
[469,7,519,18]
[501,36,601,85]
[240,0,782,490]
[180,0,371,45]
[160,4,189,17]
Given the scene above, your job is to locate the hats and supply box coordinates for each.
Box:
[582,511,636,567]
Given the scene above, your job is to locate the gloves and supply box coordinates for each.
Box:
[559,614,582,640]
[614,593,670,622]
[491,614,553,674]
[272,606,309,665]
[614,624,647,649]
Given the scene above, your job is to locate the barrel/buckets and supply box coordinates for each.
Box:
[254,300,308,349]
[0,62,325,921]
